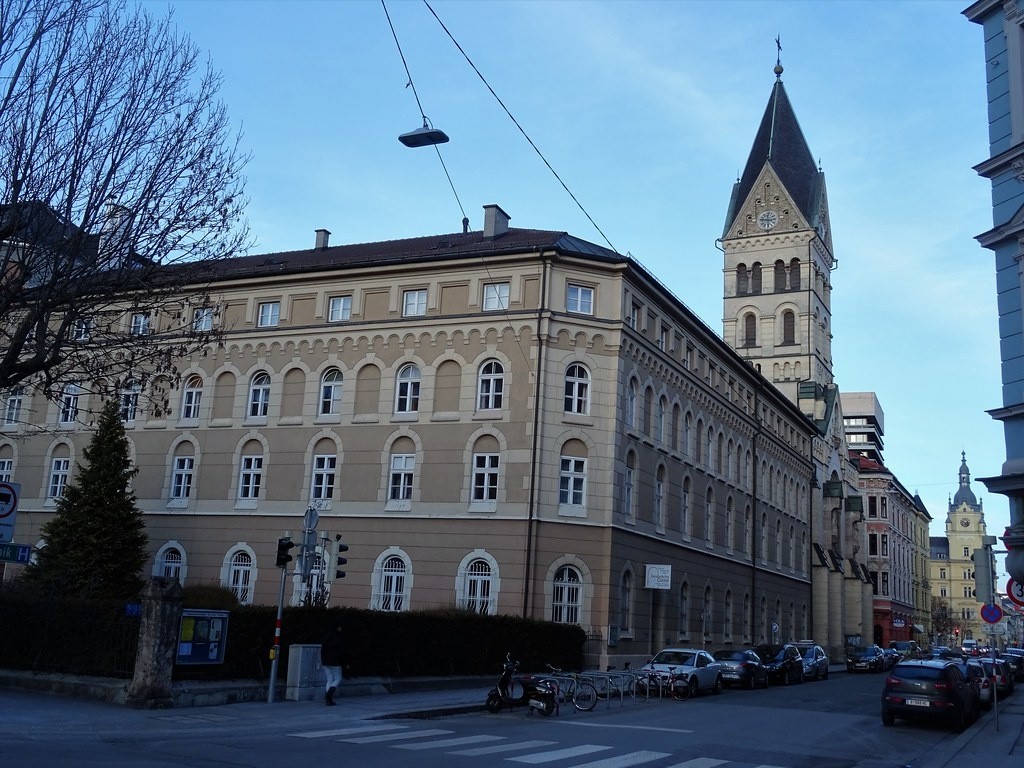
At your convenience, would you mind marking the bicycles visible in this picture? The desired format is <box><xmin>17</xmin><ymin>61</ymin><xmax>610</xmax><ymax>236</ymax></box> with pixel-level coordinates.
<box><xmin>543</xmin><ymin>658</ymin><xmax>598</xmax><ymax>713</ymax></box>
<box><xmin>638</xmin><ymin>663</ymin><xmax>692</xmax><ymax>702</ymax></box>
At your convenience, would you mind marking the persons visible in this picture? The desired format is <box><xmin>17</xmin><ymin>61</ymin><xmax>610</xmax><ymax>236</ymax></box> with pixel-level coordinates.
<box><xmin>320</xmin><ymin>622</ymin><xmax>350</xmax><ymax>706</ymax></box>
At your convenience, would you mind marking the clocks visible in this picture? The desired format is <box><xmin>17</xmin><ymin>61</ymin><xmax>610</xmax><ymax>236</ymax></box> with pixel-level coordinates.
<box><xmin>757</xmin><ymin>210</ymin><xmax>779</xmax><ymax>230</ymax></box>
<box><xmin>818</xmin><ymin>221</ymin><xmax>825</xmax><ymax>239</ymax></box>
<box><xmin>960</xmin><ymin>517</ymin><xmax>970</xmax><ymax>527</ymax></box>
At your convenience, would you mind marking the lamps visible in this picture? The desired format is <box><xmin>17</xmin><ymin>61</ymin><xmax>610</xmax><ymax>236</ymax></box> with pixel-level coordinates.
<box><xmin>813</xmin><ymin>543</ymin><xmax>831</xmax><ymax>568</ymax></box>
<box><xmin>860</xmin><ymin>563</ymin><xmax>876</xmax><ymax>586</ymax></box>
<box><xmin>848</xmin><ymin>559</ymin><xmax>865</xmax><ymax>580</ymax></box>
<box><xmin>828</xmin><ymin>549</ymin><xmax>845</xmax><ymax>574</ymax></box>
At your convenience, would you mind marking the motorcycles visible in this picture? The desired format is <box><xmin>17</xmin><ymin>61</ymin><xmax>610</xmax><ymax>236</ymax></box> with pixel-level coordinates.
<box><xmin>485</xmin><ymin>651</ymin><xmax>553</xmax><ymax>720</ymax></box>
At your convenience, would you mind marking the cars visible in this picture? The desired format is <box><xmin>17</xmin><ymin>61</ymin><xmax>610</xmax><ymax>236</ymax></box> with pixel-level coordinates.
<box><xmin>791</xmin><ymin>638</ymin><xmax>831</xmax><ymax>680</ymax></box>
<box><xmin>713</xmin><ymin>647</ymin><xmax>770</xmax><ymax>688</ymax></box>
<box><xmin>638</xmin><ymin>644</ymin><xmax>724</xmax><ymax>699</ymax></box>
<box><xmin>756</xmin><ymin>642</ymin><xmax>806</xmax><ymax>685</ymax></box>
<box><xmin>845</xmin><ymin>640</ymin><xmax>1024</xmax><ymax>732</ymax></box>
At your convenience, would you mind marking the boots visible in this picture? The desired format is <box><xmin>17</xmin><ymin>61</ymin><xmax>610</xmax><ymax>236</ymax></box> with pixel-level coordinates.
<box><xmin>325</xmin><ymin>686</ymin><xmax>336</xmax><ymax>706</ymax></box>
